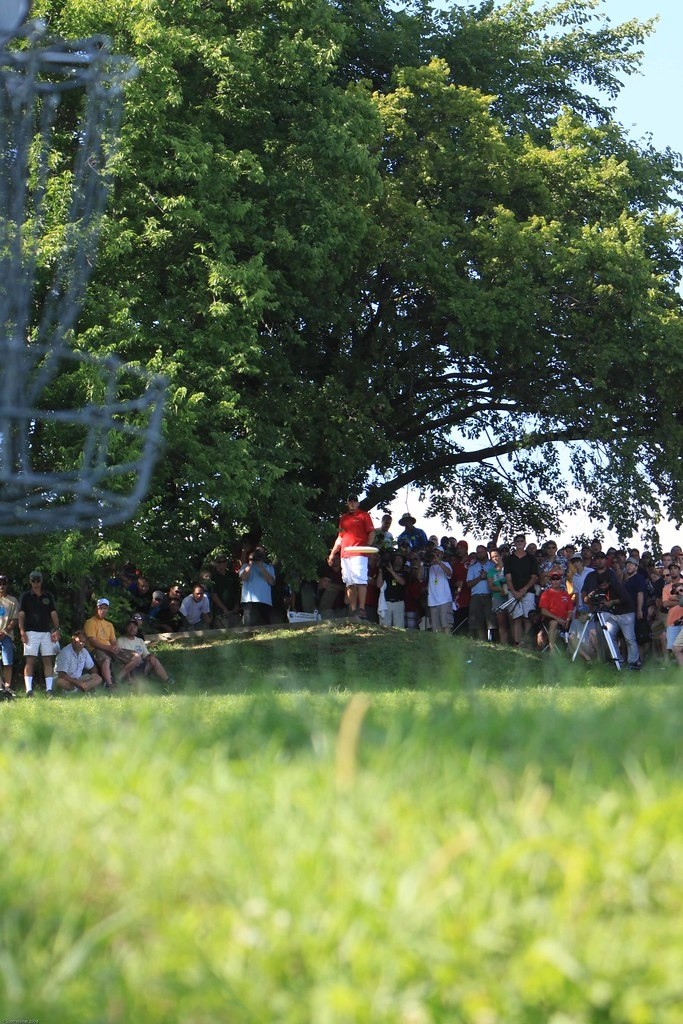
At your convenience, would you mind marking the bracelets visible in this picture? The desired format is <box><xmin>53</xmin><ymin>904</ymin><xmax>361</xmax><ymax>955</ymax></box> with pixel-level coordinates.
<box><xmin>438</xmin><ymin>562</ymin><xmax>442</xmax><ymax>565</ymax></box>
<box><xmin>610</xmin><ymin>600</ymin><xmax>614</xmax><ymax>605</ymax></box>
<box><xmin>379</xmin><ymin>571</ymin><xmax>382</xmax><ymax>573</ymax></box>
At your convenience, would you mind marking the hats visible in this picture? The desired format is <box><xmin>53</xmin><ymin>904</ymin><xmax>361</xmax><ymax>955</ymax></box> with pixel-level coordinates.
<box><xmin>550</xmin><ymin>571</ymin><xmax>561</xmax><ymax>578</ymax></box>
<box><xmin>514</xmin><ymin>535</ymin><xmax>525</xmax><ymax>541</ymax></box>
<box><xmin>625</xmin><ymin>557</ymin><xmax>637</xmax><ymax>565</ymax></box>
<box><xmin>393</xmin><ymin>549</ymin><xmax>406</xmax><ymax>560</ymax></box>
<box><xmin>670</xmin><ymin>583</ymin><xmax>683</xmax><ymax>595</ymax></box>
<box><xmin>570</xmin><ymin>554</ymin><xmax>583</xmax><ymax>561</ymax></box>
<box><xmin>0</xmin><ymin>575</ymin><xmax>9</xmax><ymax>582</ymax></box>
<box><xmin>399</xmin><ymin>513</ymin><xmax>416</xmax><ymax>526</ymax></box>
<box><xmin>594</xmin><ymin>551</ymin><xmax>607</xmax><ymax>558</ymax></box>
<box><xmin>97</xmin><ymin>598</ymin><xmax>109</xmax><ymax>607</ymax></box>
<box><xmin>432</xmin><ymin>546</ymin><xmax>444</xmax><ymax>553</ymax></box>
<box><xmin>30</xmin><ymin>571</ymin><xmax>42</xmax><ymax>579</ymax></box>
<box><xmin>348</xmin><ymin>495</ymin><xmax>358</xmax><ymax>502</ymax></box>
<box><xmin>126</xmin><ymin>618</ymin><xmax>138</xmax><ymax>626</ymax></box>
<box><xmin>668</xmin><ymin>561</ymin><xmax>681</xmax><ymax>570</ymax></box>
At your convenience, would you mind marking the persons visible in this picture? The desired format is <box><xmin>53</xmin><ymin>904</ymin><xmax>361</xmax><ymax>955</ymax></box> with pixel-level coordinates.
<box><xmin>83</xmin><ymin>598</ymin><xmax>142</xmax><ymax>692</ymax></box>
<box><xmin>447</xmin><ymin>533</ymin><xmax>683</xmax><ymax>672</ymax></box>
<box><xmin>133</xmin><ymin>611</ymin><xmax>145</xmax><ymax>640</ymax></box>
<box><xmin>107</xmin><ymin>567</ymin><xmax>232</xmax><ymax>633</ymax></box>
<box><xmin>17</xmin><ymin>571</ymin><xmax>61</xmax><ymax>698</ymax></box>
<box><xmin>53</xmin><ymin>630</ymin><xmax>103</xmax><ymax>693</ymax></box>
<box><xmin>328</xmin><ymin>494</ymin><xmax>376</xmax><ymax>619</ymax></box>
<box><xmin>115</xmin><ymin>618</ymin><xmax>176</xmax><ymax>687</ymax></box>
<box><xmin>0</xmin><ymin>574</ymin><xmax>21</xmax><ymax>695</ymax></box>
<box><xmin>238</xmin><ymin>549</ymin><xmax>276</xmax><ymax>626</ymax></box>
<box><xmin>312</xmin><ymin>552</ymin><xmax>350</xmax><ymax>620</ymax></box>
<box><xmin>345</xmin><ymin>512</ymin><xmax>455</xmax><ymax>635</ymax></box>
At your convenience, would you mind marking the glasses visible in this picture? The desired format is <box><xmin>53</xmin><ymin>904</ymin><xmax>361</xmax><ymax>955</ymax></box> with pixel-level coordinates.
<box><xmin>676</xmin><ymin>589</ymin><xmax>683</xmax><ymax>595</ymax></box>
<box><xmin>547</xmin><ymin>547</ymin><xmax>556</xmax><ymax>549</ymax></box>
<box><xmin>0</xmin><ymin>582</ymin><xmax>7</xmax><ymax>585</ymax></box>
<box><xmin>131</xmin><ymin>616</ymin><xmax>142</xmax><ymax>620</ymax></box>
<box><xmin>551</xmin><ymin>577</ymin><xmax>560</xmax><ymax>580</ymax></box>
<box><xmin>481</xmin><ymin>565</ymin><xmax>484</xmax><ymax>571</ymax></box>
<box><xmin>662</xmin><ymin>574</ymin><xmax>671</xmax><ymax>578</ymax></box>
<box><xmin>612</xmin><ymin>561</ymin><xmax>618</xmax><ymax>563</ymax></box>
<box><xmin>30</xmin><ymin>578</ymin><xmax>42</xmax><ymax>582</ymax></box>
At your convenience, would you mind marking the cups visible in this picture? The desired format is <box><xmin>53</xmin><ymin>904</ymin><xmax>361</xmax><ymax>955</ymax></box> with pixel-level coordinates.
<box><xmin>487</xmin><ymin>627</ymin><xmax>496</xmax><ymax>642</ymax></box>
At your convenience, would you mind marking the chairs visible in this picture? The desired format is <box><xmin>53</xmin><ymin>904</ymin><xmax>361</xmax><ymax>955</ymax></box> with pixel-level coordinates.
<box><xmin>74</xmin><ymin>629</ymin><xmax>122</xmax><ymax>693</ymax></box>
<box><xmin>534</xmin><ymin>595</ymin><xmax>572</xmax><ymax>654</ymax></box>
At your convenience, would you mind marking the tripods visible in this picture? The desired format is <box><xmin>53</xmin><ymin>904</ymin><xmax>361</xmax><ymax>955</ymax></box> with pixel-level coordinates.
<box><xmin>572</xmin><ymin>602</ymin><xmax>622</xmax><ymax>671</ymax></box>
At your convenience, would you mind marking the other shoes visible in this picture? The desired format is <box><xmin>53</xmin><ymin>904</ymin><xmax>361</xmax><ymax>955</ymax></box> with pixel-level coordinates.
<box><xmin>46</xmin><ymin>690</ymin><xmax>57</xmax><ymax>700</ymax></box>
<box><xmin>26</xmin><ymin>690</ymin><xmax>33</xmax><ymax>698</ymax></box>
<box><xmin>359</xmin><ymin>608</ymin><xmax>368</xmax><ymax>619</ymax></box>
<box><xmin>348</xmin><ymin>609</ymin><xmax>358</xmax><ymax>616</ymax></box>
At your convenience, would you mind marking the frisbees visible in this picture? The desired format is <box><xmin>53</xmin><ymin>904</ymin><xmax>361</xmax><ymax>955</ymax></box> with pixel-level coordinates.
<box><xmin>343</xmin><ymin>546</ymin><xmax>379</xmax><ymax>553</ymax></box>
